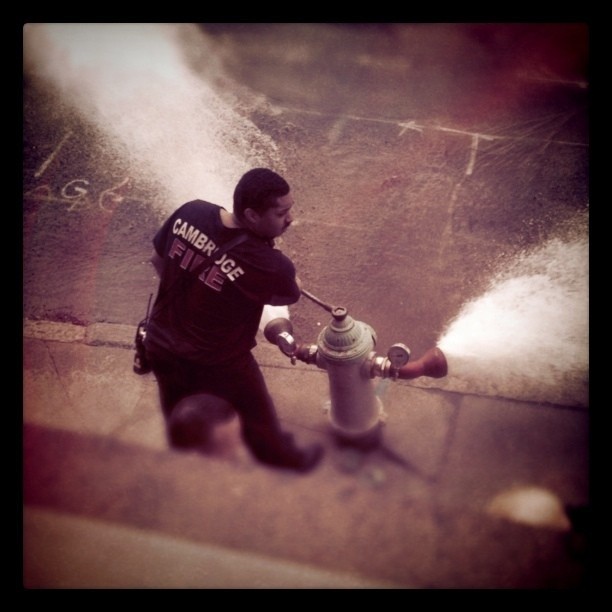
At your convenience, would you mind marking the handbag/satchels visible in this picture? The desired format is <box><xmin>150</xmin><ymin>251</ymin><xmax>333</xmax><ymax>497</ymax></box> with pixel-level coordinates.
<box><xmin>133</xmin><ymin>233</ymin><xmax>275</xmax><ymax>374</ymax></box>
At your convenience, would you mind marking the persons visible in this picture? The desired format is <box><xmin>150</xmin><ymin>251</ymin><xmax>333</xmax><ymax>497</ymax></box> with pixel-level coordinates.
<box><xmin>143</xmin><ymin>168</ymin><xmax>320</xmax><ymax>471</ymax></box>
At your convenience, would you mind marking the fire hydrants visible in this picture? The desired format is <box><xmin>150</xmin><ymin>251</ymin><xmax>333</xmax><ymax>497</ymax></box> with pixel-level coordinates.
<box><xmin>278</xmin><ymin>291</ymin><xmax>449</xmax><ymax>451</ymax></box>
